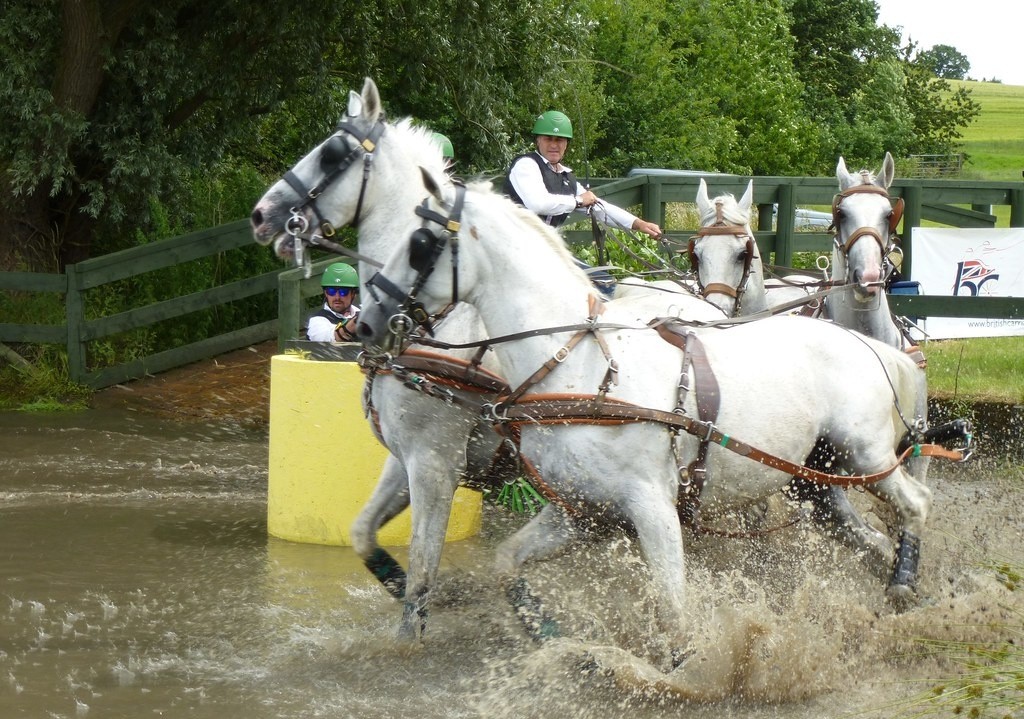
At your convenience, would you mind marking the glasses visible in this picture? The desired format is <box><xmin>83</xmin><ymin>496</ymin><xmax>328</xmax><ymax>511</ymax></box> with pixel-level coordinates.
<box><xmin>324</xmin><ymin>287</ymin><xmax>353</xmax><ymax>296</ymax></box>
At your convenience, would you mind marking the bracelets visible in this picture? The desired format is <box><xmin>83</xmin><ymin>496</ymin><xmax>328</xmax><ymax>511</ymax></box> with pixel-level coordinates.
<box><xmin>574</xmin><ymin>195</ymin><xmax>583</xmax><ymax>207</ymax></box>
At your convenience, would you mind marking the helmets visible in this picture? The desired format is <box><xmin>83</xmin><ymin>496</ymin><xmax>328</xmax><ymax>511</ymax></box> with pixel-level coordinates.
<box><xmin>320</xmin><ymin>263</ymin><xmax>359</xmax><ymax>288</ymax></box>
<box><xmin>431</xmin><ymin>133</ymin><xmax>454</xmax><ymax>159</ymax></box>
<box><xmin>532</xmin><ymin>111</ymin><xmax>573</xmax><ymax>139</ymax></box>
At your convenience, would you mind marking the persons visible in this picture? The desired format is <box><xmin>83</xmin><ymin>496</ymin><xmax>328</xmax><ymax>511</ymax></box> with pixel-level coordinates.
<box><xmin>308</xmin><ymin>263</ymin><xmax>358</xmax><ymax>342</ymax></box>
<box><xmin>505</xmin><ymin>111</ymin><xmax>663</xmax><ymax>240</ymax></box>
<box><xmin>432</xmin><ymin>132</ymin><xmax>453</xmax><ymax>172</ymax></box>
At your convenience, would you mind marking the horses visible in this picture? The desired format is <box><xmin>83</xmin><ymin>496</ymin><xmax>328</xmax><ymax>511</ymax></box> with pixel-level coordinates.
<box><xmin>684</xmin><ymin>175</ymin><xmax>828</xmax><ymax>335</ymax></box>
<box><xmin>826</xmin><ymin>152</ymin><xmax>927</xmax><ymax>401</ymax></box>
<box><xmin>351</xmin><ymin>166</ymin><xmax>933</xmax><ymax>659</ymax></box>
<box><xmin>251</xmin><ymin>73</ymin><xmax>529</xmax><ymax>653</ymax></box>
